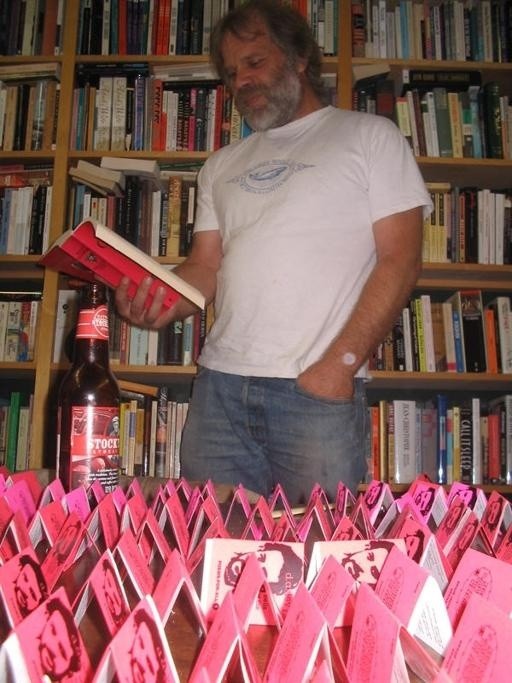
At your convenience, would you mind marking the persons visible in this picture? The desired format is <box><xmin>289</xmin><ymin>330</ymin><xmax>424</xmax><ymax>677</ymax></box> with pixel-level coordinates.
<box><xmin>38</xmin><ymin>597</ymin><xmax>82</xmax><ymax>680</ymax></box>
<box><xmin>10</xmin><ymin>551</ymin><xmax>50</xmax><ymax>615</ymax></box>
<box><xmin>126</xmin><ymin>606</ymin><xmax>168</xmax><ymax>681</ymax></box>
<box><xmin>334</xmin><ymin>538</ymin><xmax>399</xmax><ymax>587</ymax></box>
<box><xmin>269</xmin><ymin>481</ymin><xmax>512</xmax><ymax>564</ymax></box>
<box><xmin>96</xmin><ymin>558</ymin><xmax>125</xmax><ymax>626</ymax></box>
<box><xmin>113</xmin><ymin>1</ymin><xmax>434</xmax><ymax>505</ymax></box>
<box><xmin>221</xmin><ymin>540</ymin><xmax>304</xmax><ymax>595</ymax></box>
<box><xmin>51</xmin><ymin>519</ymin><xmax>85</xmax><ymax>569</ymax></box>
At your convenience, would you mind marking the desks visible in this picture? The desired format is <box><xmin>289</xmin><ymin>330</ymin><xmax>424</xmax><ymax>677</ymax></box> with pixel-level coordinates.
<box><xmin>0</xmin><ymin>484</ymin><xmax>511</xmax><ymax>682</ymax></box>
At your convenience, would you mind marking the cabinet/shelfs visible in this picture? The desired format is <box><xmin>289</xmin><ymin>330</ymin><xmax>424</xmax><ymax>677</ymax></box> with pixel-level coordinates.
<box><xmin>0</xmin><ymin>2</ymin><xmax>79</xmax><ymax>475</ymax></box>
<box><xmin>41</xmin><ymin>1</ymin><xmax>352</xmax><ymax>493</ymax></box>
<box><xmin>340</xmin><ymin>1</ymin><xmax>512</xmax><ymax>500</ymax></box>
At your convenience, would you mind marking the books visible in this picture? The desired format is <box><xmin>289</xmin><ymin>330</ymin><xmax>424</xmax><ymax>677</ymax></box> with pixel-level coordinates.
<box><xmin>254</xmin><ymin>501</ymin><xmax>350</xmax><ymax>518</ymax></box>
<box><xmin>1</xmin><ymin>0</ymin><xmax>510</xmax><ymax>488</ymax></box>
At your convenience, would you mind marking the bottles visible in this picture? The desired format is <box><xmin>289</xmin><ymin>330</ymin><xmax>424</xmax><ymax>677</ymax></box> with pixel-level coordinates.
<box><xmin>51</xmin><ymin>280</ymin><xmax>123</xmax><ymax>513</ymax></box>
